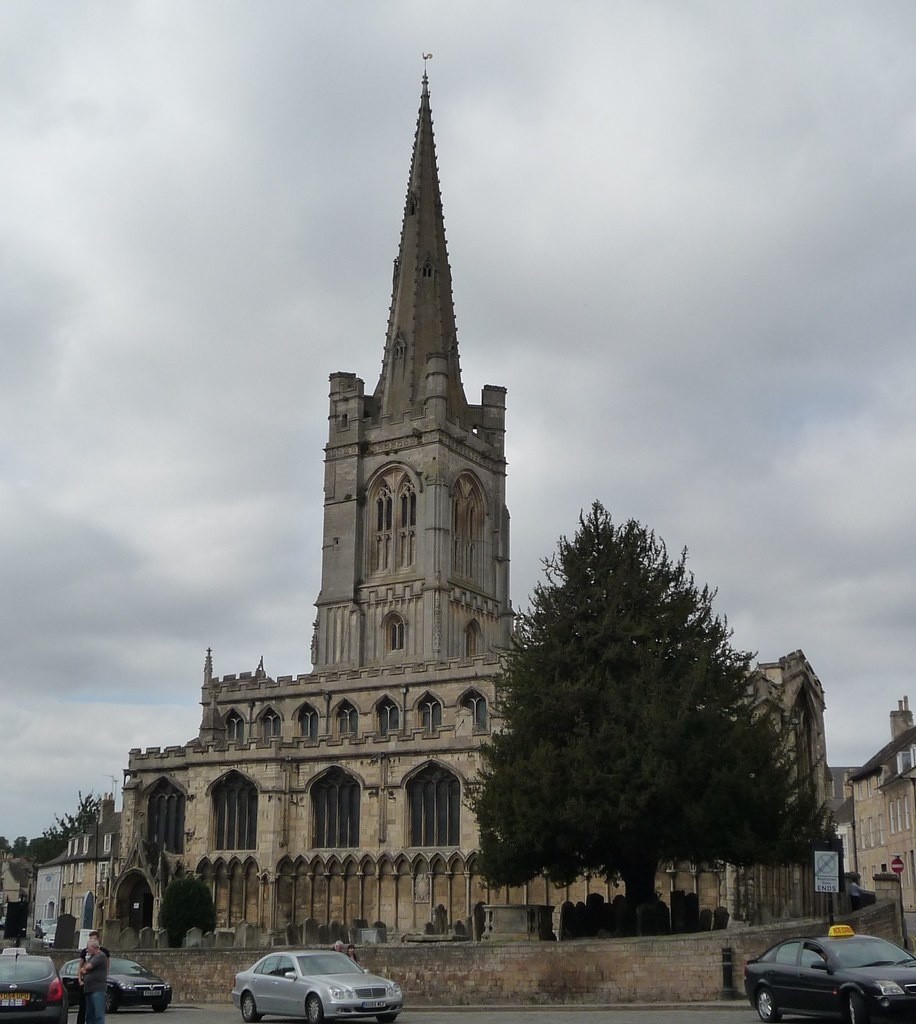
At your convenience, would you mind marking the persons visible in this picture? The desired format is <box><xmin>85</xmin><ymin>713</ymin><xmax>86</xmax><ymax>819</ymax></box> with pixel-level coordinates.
<box><xmin>77</xmin><ymin>931</ymin><xmax>110</xmax><ymax>1024</ymax></box>
<box><xmin>331</xmin><ymin>940</ymin><xmax>357</xmax><ymax>964</ymax></box>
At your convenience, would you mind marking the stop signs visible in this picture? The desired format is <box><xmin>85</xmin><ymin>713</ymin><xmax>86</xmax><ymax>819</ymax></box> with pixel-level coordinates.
<box><xmin>890</xmin><ymin>858</ymin><xmax>905</xmax><ymax>874</ymax></box>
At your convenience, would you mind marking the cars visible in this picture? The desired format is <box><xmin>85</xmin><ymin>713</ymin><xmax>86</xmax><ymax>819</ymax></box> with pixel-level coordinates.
<box><xmin>745</xmin><ymin>923</ymin><xmax>916</xmax><ymax>1024</ymax></box>
<box><xmin>230</xmin><ymin>950</ymin><xmax>405</xmax><ymax>1024</ymax></box>
<box><xmin>58</xmin><ymin>954</ymin><xmax>172</xmax><ymax>1013</ymax></box>
<box><xmin>0</xmin><ymin>947</ymin><xmax>69</xmax><ymax>1024</ymax></box>
<box><xmin>41</xmin><ymin>924</ymin><xmax>57</xmax><ymax>946</ymax></box>
